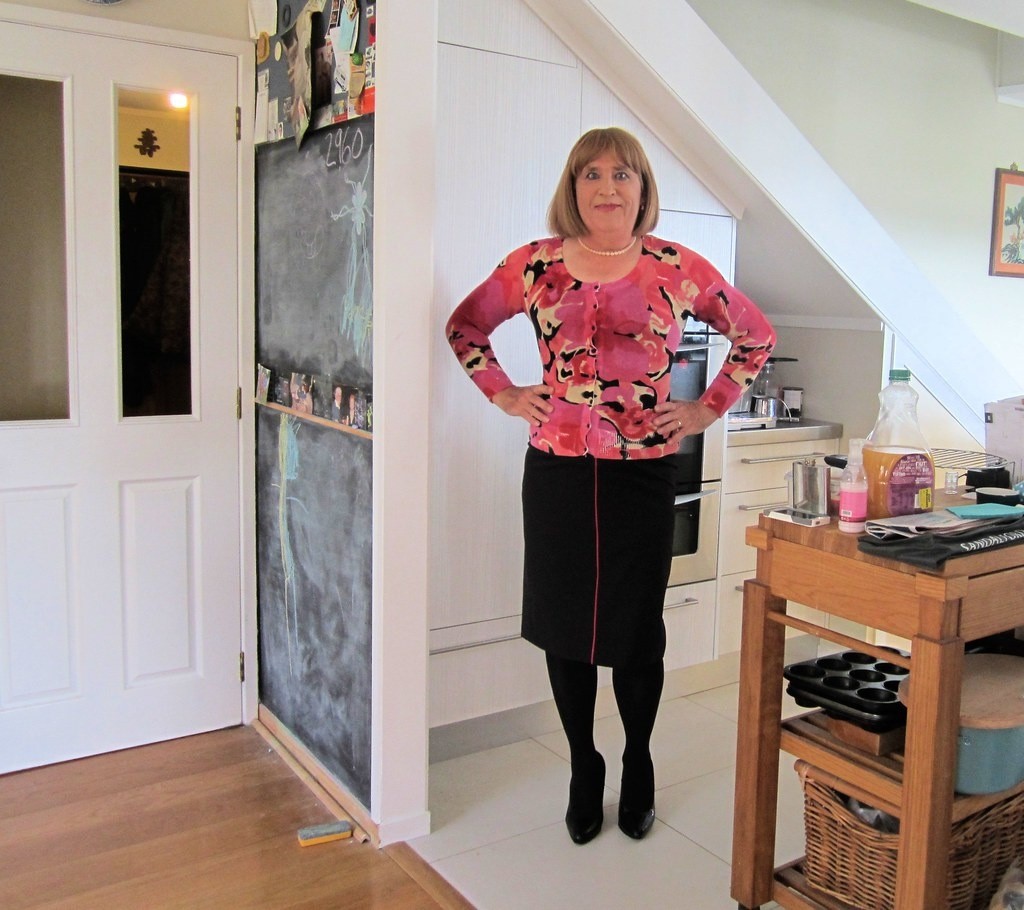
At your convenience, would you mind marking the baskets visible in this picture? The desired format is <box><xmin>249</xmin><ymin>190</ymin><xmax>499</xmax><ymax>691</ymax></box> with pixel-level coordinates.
<box><xmin>803</xmin><ymin>780</ymin><xmax>1024</xmax><ymax>910</ymax></box>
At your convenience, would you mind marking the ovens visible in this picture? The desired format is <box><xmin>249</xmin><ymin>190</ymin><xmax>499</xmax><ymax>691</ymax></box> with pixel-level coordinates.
<box><xmin>666</xmin><ymin>332</ymin><xmax>729</xmax><ymax>589</ymax></box>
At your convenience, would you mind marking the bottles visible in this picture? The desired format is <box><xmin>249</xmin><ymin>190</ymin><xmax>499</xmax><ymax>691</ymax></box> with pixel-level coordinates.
<box><xmin>751</xmin><ymin>361</ymin><xmax>781</xmax><ymax>418</ymax></box>
<box><xmin>860</xmin><ymin>369</ymin><xmax>935</xmax><ymax>520</ymax></box>
<box><xmin>838</xmin><ymin>439</ymin><xmax>868</xmax><ymax>534</ymax></box>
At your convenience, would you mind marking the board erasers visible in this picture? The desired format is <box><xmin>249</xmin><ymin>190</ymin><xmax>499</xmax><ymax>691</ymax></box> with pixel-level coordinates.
<box><xmin>297</xmin><ymin>820</ymin><xmax>352</xmax><ymax>847</ymax></box>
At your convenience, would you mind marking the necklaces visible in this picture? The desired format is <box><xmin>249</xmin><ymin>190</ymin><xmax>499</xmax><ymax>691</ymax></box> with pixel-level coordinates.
<box><xmin>577</xmin><ymin>236</ymin><xmax>637</xmax><ymax>256</ymax></box>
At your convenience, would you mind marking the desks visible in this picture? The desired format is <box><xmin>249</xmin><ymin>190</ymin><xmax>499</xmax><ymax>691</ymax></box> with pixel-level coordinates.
<box><xmin>731</xmin><ymin>487</ymin><xmax>1024</xmax><ymax>910</ymax></box>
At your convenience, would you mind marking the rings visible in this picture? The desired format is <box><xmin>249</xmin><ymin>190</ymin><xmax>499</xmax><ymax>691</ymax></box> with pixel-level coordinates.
<box><xmin>677</xmin><ymin>420</ymin><xmax>683</xmax><ymax>429</ymax></box>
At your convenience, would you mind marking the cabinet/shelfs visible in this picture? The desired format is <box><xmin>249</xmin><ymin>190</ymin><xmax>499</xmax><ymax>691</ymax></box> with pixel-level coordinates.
<box><xmin>718</xmin><ymin>439</ymin><xmax>841</xmax><ymax>657</ymax></box>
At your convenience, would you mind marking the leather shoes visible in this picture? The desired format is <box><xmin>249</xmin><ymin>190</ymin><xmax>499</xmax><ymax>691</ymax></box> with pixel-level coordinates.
<box><xmin>618</xmin><ymin>753</ymin><xmax>654</xmax><ymax>839</ymax></box>
<box><xmin>567</xmin><ymin>752</ymin><xmax>605</xmax><ymax>844</ymax></box>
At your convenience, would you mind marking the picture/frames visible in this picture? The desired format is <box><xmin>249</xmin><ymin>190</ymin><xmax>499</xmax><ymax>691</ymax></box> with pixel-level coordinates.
<box><xmin>987</xmin><ymin>168</ymin><xmax>1024</xmax><ymax>278</ymax></box>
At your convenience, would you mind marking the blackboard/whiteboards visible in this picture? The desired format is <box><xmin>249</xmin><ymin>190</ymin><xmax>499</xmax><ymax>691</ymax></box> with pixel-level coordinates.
<box><xmin>252</xmin><ymin>112</ymin><xmax>375</xmax><ymax>440</ymax></box>
<box><xmin>255</xmin><ymin>402</ymin><xmax>373</xmax><ymax>815</ymax></box>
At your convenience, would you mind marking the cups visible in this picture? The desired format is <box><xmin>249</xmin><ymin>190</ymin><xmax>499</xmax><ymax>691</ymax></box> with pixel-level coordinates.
<box><xmin>975</xmin><ymin>487</ymin><xmax>1019</xmax><ymax>507</ymax></box>
<box><xmin>754</xmin><ymin>398</ymin><xmax>777</xmax><ymax>417</ymax></box>
<box><xmin>966</xmin><ymin>467</ymin><xmax>1010</xmax><ymax>489</ymax></box>
<box><xmin>793</xmin><ymin>461</ymin><xmax>827</xmax><ymax>516</ymax></box>
<box><xmin>944</xmin><ymin>471</ymin><xmax>959</xmax><ymax>494</ymax></box>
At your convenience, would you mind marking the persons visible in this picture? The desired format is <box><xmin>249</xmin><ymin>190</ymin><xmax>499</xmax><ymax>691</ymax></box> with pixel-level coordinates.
<box><xmin>446</xmin><ymin>128</ymin><xmax>777</xmax><ymax>846</ymax></box>
